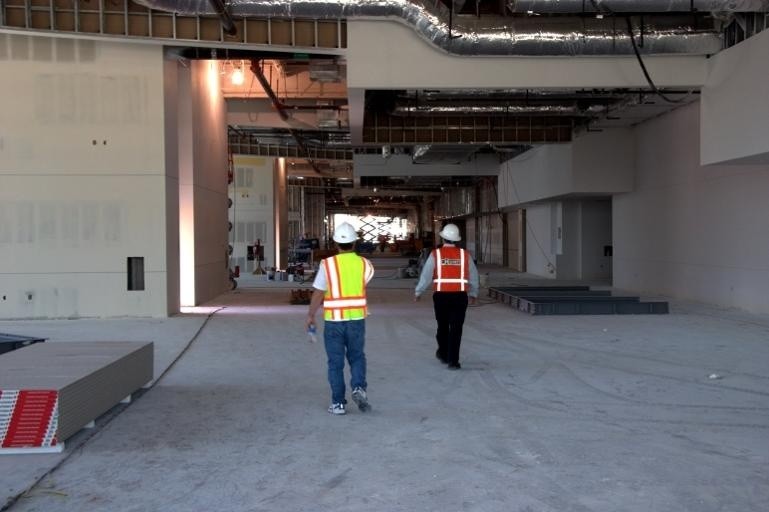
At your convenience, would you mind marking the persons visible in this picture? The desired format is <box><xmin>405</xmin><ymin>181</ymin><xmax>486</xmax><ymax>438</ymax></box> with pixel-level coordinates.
<box><xmin>413</xmin><ymin>223</ymin><xmax>481</xmax><ymax>369</ymax></box>
<box><xmin>303</xmin><ymin>222</ymin><xmax>375</xmax><ymax>415</ymax></box>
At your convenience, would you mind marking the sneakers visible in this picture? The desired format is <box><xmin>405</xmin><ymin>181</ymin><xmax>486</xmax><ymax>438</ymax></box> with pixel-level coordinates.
<box><xmin>351</xmin><ymin>386</ymin><xmax>372</xmax><ymax>412</ymax></box>
<box><xmin>327</xmin><ymin>402</ymin><xmax>346</xmax><ymax>414</ymax></box>
<box><xmin>436</xmin><ymin>352</ymin><xmax>460</xmax><ymax>370</ymax></box>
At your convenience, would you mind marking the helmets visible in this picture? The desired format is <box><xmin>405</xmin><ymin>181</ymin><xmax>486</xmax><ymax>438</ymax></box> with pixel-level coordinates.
<box><xmin>439</xmin><ymin>224</ymin><xmax>462</xmax><ymax>241</ymax></box>
<box><xmin>333</xmin><ymin>222</ymin><xmax>361</xmax><ymax>244</ymax></box>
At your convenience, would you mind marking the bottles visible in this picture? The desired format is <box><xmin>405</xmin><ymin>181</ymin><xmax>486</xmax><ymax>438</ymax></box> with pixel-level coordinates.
<box><xmin>307</xmin><ymin>321</ymin><xmax>318</xmax><ymax>344</ymax></box>
